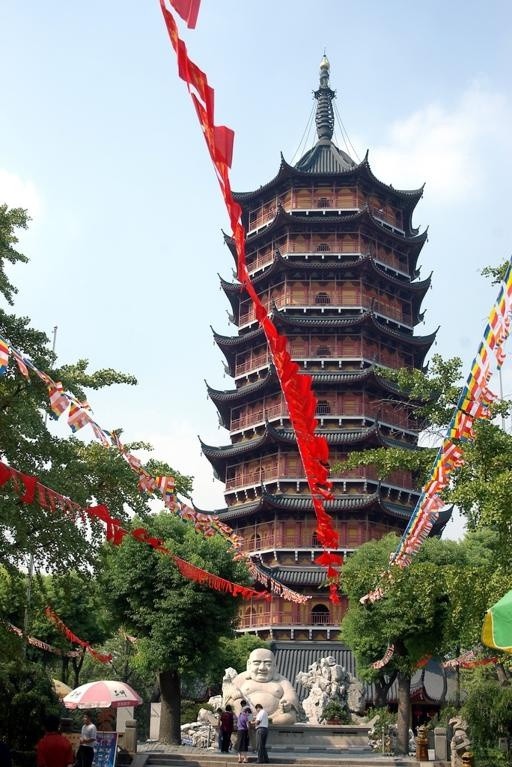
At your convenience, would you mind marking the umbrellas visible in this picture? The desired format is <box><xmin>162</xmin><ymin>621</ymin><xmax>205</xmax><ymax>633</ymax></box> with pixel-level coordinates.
<box><xmin>62</xmin><ymin>679</ymin><xmax>146</xmax><ymax>737</ymax></box>
<box><xmin>48</xmin><ymin>675</ymin><xmax>75</xmax><ymax>700</ymax></box>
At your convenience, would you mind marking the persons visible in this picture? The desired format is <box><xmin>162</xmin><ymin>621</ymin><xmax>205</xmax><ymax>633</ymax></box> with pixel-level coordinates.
<box><xmin>72</xmin><ymin>708</ymin><xmax>99</xmax><ymax>766</ymax></box>
<box><xmin>238</xmin><ymin>699</ymin><xmax>254</xmax><ymax>749</ymax></box>
<box><xmin>219</xmin><ymin>704</ymin><xmax>234</xmax><ymax>752</ymax></box>
<box><xmin>249</xmin><ymin>703</ymin><xmax>271</xmax><ymax>763</ymax></box>
<box><xmin>36</xmin><ymin>713</ymin><xmax>77</xmax><ymax>766</ymax></box>
<box><xmin>216</xmin><ymin>708</ymin><xmax>234</xmax><ymax>751</ymax></box>
<box><xmin>225</xmin><ymin>646</ymin><xmax>300</xmax><ymax>724</ymax></box>
<box><xmin>237</xmin><ymin>707</ymin><xmax>253</xmax><ymax>763</ymax></box>
